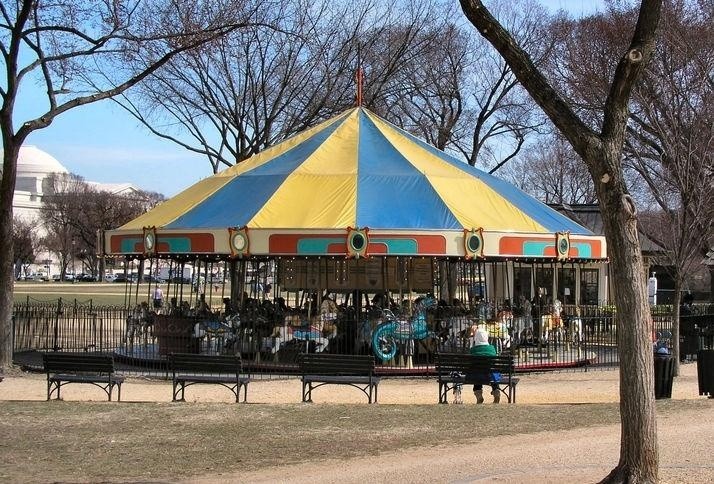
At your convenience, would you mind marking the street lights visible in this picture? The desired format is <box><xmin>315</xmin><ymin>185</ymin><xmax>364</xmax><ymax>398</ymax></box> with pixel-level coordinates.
<box><xmin>71</xmin><ymin>233</ymin><xmax>76</xmax><ymax>276</ymax></box>
<box><xmin>80</xmin><ymin>248</ymin><xmax>87</xmax><ymax>274</ymax></box>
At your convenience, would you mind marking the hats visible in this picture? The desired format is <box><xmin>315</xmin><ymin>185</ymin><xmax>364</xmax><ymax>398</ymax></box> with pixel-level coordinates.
<box><xmin>475</xmin><ymin>327</ymin><xmax>489</xmax><ymax>346</ymax></box>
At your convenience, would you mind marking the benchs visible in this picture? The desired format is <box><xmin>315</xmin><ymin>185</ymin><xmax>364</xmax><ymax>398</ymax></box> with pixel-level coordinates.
<box><xmin>434</xmin><ymin>351</ymin><xmax>520</xmax><ymax>404</ymax></box>
<box><xmin>296</xmin><ymin>350</ymin><xmax>381</xmax><ymax>404</ymax></box>
<box><xmin>168</xmin><ymin>350</ymin><xmax>251</xmax><ymax>403</ymax></box>
<box><xmin>41</xmin><ymin>349</ymin><xmax>125</xmax><ymax>401</ymax></box>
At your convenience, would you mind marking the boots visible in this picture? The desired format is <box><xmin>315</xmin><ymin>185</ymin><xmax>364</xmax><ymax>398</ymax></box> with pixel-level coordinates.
<box><xmin>491</xmin><ymin>389</ymin><xmax>501</xmax><ymax>404</ymax></box>
<box><xmin>474</xmin><ymin>389</ymin><xmax>484</xmax><ymax>404</ymax></box>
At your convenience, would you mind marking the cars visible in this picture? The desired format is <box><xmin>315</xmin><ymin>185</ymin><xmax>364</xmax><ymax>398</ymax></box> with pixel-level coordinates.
<box><xmin>17</xmin><ymin>261</ymin><xmax>278</xmax><ymax>284</ymax></box>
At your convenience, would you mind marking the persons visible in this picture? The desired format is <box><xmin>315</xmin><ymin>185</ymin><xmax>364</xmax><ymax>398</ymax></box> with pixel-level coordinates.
<box><xmin>265</xmin><ymin>279</ymin><xmax>272</xmax><ymax>302</ymax></box>
<box><xmin>197</xmin><ymin>273</ymin><xmax>204</xmax><ymax>292</ymax></box>
<box><xmin>150</xmin><ymin>282</ymin><xmax>164</xmax><ymax>314</ymax></box>
<box><xmin>192</xmin><ymin>274</ymin><xmax>198</xmax><ymax>292</ymax></box>
<box><xmin>464</xmin><ymin>326</ymin><xmax>500</xmax><ymax>403</ymax></box>
<box><xmin>252</xmin><ymin>278</ymin><xmax>263</xmax><ymax>298</ymax></box>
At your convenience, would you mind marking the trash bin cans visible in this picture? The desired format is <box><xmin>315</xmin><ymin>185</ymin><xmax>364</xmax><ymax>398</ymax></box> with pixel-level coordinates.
<box><xmin>697</xmin><ymin>349</ymin><xmax>714</xmax><ymax>399</ymax></box>
<box><xmin>653</xmin><ymin>353</ymin><xmax>677</xmax><ymax>400</ymax></box>
